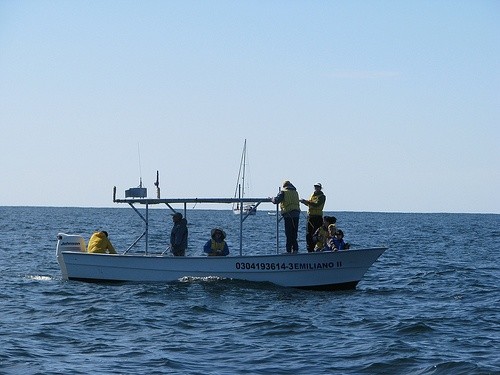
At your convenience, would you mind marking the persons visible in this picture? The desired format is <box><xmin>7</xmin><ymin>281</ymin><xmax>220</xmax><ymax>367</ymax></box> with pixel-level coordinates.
<box><xmin>169</xmin><ymin>213</ymin><xmax>188</xmax><ymax>256</ymax></box>
<box><xmin>202</xmin><ymin>226</ymin><xmax>230</xmax><ymax>256</ymax></box>
<box><xmin>271</xmin><ymin>180</ymin><xmax>301</xmax><ymax>253</ymax></box>
<box><xmin>88</xmin><ymin>231</ymin><xmax>117</xmax><ymax>254</ymax></box>
<box><xmin>302</xmin><ymin>183</ymin><xmax>326</xmax><ymax>253</ymax></box>
<box><xmin>313</xmin><ymin>217</ymin><xmax>349</xmax><ymax>251</ymax></box>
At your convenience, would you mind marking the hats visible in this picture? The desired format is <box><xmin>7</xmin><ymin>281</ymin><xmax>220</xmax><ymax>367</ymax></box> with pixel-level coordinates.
<box><xmin>314</xmin><ymin>183</ymin><xmax>322</xmax><ymax>189</ymax></box>
<box><xmin>211</xmin><ymin>228</ymin><xmax>226</xmax><ymax>239</ymax></box>
<box><xmin>170</xmin><ymin>213</ymin><xmax>183</xmax><ymax>220</ymax></box>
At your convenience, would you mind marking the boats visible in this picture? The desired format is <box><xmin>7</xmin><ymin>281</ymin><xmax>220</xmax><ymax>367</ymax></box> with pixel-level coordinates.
<box><xmin>55</xmin><ymin>232</ymin><xmax>388</xmax><ymax>288</ymax></box>
